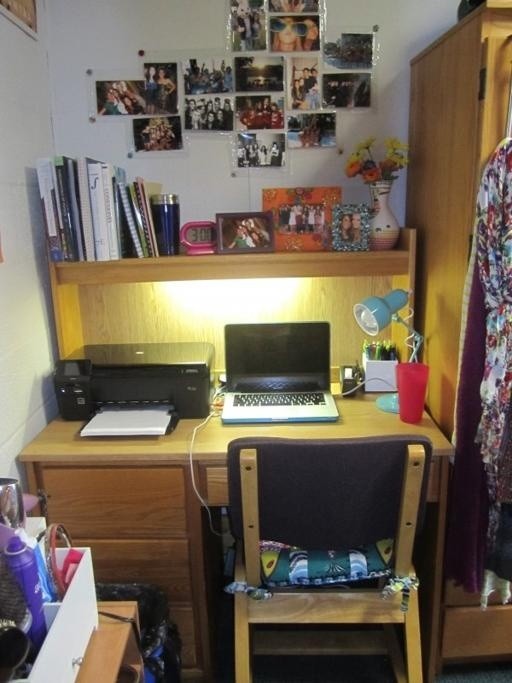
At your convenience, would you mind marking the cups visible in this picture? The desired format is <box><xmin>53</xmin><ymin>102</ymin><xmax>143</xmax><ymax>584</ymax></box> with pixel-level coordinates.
<box><xmin>397</xmin><ymin>361</ymin><xmax>430</xmax><ymax>425</ymax></box>
<box><xmin>149</xmin><ymin>193</ymin><xmax>181</xmax><ymax>256</ymax></box>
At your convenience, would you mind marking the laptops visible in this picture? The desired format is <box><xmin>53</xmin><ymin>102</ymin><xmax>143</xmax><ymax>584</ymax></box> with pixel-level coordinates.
<box><xmin>220</xmin><ymin>322</ymin><xmax>340</xmax><ymax>424</ymax></box>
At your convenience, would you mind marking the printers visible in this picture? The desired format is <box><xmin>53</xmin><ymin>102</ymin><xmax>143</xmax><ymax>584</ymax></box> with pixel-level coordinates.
<box><xmin>54</xmin><ymin>342</ymin><xmax>216</xmax><ymax>437</ymax></box>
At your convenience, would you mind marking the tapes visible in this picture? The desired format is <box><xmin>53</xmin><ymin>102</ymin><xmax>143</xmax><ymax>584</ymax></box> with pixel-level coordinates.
<box><xmin>344</xmin><ymin>367</ymin><xmax>353</xmax><ymax>379</ymax></box>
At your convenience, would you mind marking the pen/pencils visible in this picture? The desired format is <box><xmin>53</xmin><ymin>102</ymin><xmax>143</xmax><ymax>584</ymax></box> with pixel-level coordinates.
<box><xmin>362</xmin><ymin>338</ymin><xmax>396</xmax><ymax>360</ymax></box>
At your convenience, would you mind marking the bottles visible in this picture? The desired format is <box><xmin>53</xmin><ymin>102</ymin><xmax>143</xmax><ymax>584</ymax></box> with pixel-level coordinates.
<box><xmin>4</xmin><ymin>537</ymin><xmax>48</xmax><ymax>649</ymax></box>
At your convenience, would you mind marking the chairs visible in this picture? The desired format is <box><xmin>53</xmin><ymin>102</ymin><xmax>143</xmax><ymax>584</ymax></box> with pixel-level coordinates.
<box><xmin>221</xmin><ymin>434</ymin><xmax>433</xmax><ymax>682</ymax></box>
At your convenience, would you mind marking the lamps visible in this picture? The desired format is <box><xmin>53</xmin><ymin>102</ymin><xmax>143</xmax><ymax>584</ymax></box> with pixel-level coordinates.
<box><xmin>352</xmin><ymin>288</ymin><xmax>425</xmax><ymax>415</ymax></box>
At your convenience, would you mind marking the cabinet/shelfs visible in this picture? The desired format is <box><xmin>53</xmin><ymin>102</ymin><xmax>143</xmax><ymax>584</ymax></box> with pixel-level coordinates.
<box><xmin>405</xmin><ymin>1</ymin><xmax>511</xmax><ymax>680</ymax></box>
<box><xmin>78</xmin><ymin>601</ymin><xmax>147</xmax><ymax>682</ymax></box>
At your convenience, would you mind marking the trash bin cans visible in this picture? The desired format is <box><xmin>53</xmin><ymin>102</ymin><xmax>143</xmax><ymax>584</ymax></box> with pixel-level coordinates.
<box><xmin>95</xmin><ymin>582</ymin><xmax>182</xmax><ymax>683</ymax></box>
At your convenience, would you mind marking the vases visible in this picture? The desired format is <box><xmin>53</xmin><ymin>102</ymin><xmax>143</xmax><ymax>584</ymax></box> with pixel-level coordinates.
<box><xmin>367</xmin><ymin>180</ymin><xmax>400</xmax><ymax>249</ymax></box>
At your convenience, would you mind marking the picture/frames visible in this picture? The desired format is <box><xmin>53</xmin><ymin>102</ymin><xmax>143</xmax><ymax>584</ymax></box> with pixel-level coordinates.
<box><xmin>331</xmin><ymin>202</ymin><xmax>370</xmax><ymax>250</ymax></box>
<box><xmin>215</xmin><ymin>211</ymin><xmax>276</xmax><ymax>254</ymax></box>
<box><xmin>261</xmin><ymin>186</ymin><xmax>342</xmax><ymax>252</ymax></box>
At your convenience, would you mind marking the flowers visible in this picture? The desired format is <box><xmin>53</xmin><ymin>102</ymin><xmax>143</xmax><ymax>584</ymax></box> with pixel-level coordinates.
<box><xmin>341</xmin><ymin>135</ymin><xmax>410</xmax><ymax>187</ymax></box>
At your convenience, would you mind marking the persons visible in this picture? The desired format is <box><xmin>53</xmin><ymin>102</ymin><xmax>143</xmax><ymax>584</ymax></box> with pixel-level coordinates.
<box><xmin>232</xmin><ymin>0</ymin><xmax>261</xmax><ymax>52</ymax></box>
<box><xmin>270</xmin><ymin>16</ymin><xmax>318</xmax><ymax>51</ymax></box>
<box><xmin>98</xmin><ymin>59</ymin><xmax>318</xmax><ymax>167</ymax></box>
<box><xmin>278</xmin><ymin>203</ymin><xmax>325</xmax><ymax>235</ymax></box>
<box><xmin>228</xmin><ymin>216</ymin><xmax>270</xmax><ymax>249</ymax></box>
<box><xmin>340</xmin><ymin>214</ymin><xmax>355</xmax><ymax>242</ymax></box>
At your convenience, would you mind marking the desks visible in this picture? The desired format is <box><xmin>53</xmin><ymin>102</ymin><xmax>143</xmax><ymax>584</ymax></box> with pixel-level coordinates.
<box><xmin>20</xmin><ymin>226</ymin><xmax>454</xmax><ymax>681</ymax></box>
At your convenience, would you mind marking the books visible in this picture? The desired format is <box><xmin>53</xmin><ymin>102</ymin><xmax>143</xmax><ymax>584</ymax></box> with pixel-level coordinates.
<box><xmin>36</xmin><ymin>154</ymin><xmax>164</xmax><ymax>263</ymax></box>
<box><xmin>80</xmin><ymin>404</ymin><xmax>175</xmax><ymax>437</ymax></box>
